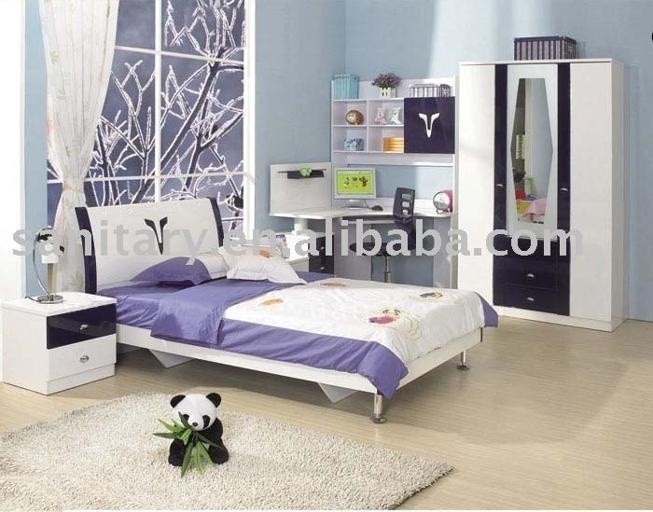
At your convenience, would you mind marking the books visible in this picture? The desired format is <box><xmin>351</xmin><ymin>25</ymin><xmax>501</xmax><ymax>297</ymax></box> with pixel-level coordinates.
<box><xmin>335</xmin><ymin>73</ymin><xmax>358</xmax><ymax>99</ymax></box>
<box><xmin>408</xmin><ymin>83</ymin><xmax>450</xmax><ymax>97</ymax></box>
<box><xmin>382</xmin><ymin>137</ymin><xmax>404</xmax><ymax>152</ymax></box>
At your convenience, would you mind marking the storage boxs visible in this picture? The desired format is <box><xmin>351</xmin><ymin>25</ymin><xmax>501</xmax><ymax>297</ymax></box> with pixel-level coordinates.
<box><xmin>514</xmin><ymin>36</ymin><xmax>577</xmax><ymax>61</ymax></box>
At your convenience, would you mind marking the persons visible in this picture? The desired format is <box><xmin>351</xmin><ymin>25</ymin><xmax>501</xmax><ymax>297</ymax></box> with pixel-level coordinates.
<box><xmin>168</xmin><ymin>392</ymin><xmax>228</xmax><ymax>466</ymax></box>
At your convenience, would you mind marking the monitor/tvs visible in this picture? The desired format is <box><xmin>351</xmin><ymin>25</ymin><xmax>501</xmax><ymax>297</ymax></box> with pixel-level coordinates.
<box><xmin>333</xmin><ymin>167</ymin><xmax>376</xmax><ymax>209</ymax></box>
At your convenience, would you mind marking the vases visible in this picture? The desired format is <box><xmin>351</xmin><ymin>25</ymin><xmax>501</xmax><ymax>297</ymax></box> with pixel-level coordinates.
<box><xmin>378</xmin><ymin>87</ymin><xmax>396</xmax><ymax>98</ymax></box>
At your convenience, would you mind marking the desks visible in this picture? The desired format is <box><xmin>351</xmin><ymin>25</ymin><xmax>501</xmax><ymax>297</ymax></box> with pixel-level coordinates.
<box><xmin>271</xmin><ymin>197</ymin><xmax>457</xmax><ymax>291</ymax></box>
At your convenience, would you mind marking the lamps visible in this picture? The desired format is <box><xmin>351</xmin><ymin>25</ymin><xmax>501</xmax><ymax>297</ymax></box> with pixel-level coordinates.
<box><xmin>24</xmin><ymin>224</ymin><xmax>65</xmax><ymax>305</ymax></box>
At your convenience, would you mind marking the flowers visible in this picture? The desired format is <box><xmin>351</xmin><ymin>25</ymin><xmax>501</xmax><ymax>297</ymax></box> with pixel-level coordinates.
<box><xmin>371</xmin><ymin>72</ymin><xmax>402</xmax><ymax>91</ymax></box>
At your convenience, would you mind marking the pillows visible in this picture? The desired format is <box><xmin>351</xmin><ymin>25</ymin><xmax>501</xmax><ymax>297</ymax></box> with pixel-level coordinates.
<box><xmin>129</xmin><ymin>241</ymin><xmax>308</xmax><ymax>294</ymax></box>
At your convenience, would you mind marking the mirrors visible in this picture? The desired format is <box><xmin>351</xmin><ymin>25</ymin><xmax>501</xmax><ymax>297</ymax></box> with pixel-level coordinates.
<box><xmin>506</xmin><ymin>64</ymin><xmax>558</xmax><ymax>240</ymax></box>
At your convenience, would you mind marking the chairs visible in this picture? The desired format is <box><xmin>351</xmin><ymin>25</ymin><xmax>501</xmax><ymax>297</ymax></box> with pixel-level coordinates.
<box><xmin>348</xmin><ymin>186</ymin><xmax>417</xmax><ymax>282</ymax></box>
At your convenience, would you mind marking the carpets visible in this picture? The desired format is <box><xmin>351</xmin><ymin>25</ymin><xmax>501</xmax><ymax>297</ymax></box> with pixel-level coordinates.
<box><xmin>0</xmin><ymin>390</ymin><xmax>455</xmax><ymax>511</ymax></box>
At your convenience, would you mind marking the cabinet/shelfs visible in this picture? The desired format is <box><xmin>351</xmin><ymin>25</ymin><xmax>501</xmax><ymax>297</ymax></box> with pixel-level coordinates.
<box><xmin>456</xmin><ymin>56</ymin><xmax>631</xmax><ymax>333</ymax></box>
<box><xmin>330</xmin><ymin>78</ymin><xmax>455</xmax><ymax>167</ymax></box>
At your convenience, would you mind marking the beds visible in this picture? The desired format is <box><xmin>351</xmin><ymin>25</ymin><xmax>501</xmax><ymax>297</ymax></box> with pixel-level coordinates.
<box><xmin>74</xmin><ymin>197</ymin><xmax>481</xmax><ymax>423</ymax></box>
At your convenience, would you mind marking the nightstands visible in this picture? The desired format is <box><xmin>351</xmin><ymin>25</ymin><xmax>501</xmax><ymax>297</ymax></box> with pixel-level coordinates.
<box><xmin>1</xmin><ymin>290</ymin><xmax>117</xmax><ymax>397</ymax></box>
<box><xmin>278</xmin><ymin>243</ymin><xmax>311</xmax><ymax>272</ymax></box>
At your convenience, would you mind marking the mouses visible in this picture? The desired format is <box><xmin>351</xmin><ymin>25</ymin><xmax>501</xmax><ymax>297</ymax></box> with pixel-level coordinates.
<box><xmin>372</xmin><ymin>205</ymin><xmax>384</xmax><ymax>211</ymax></box>
